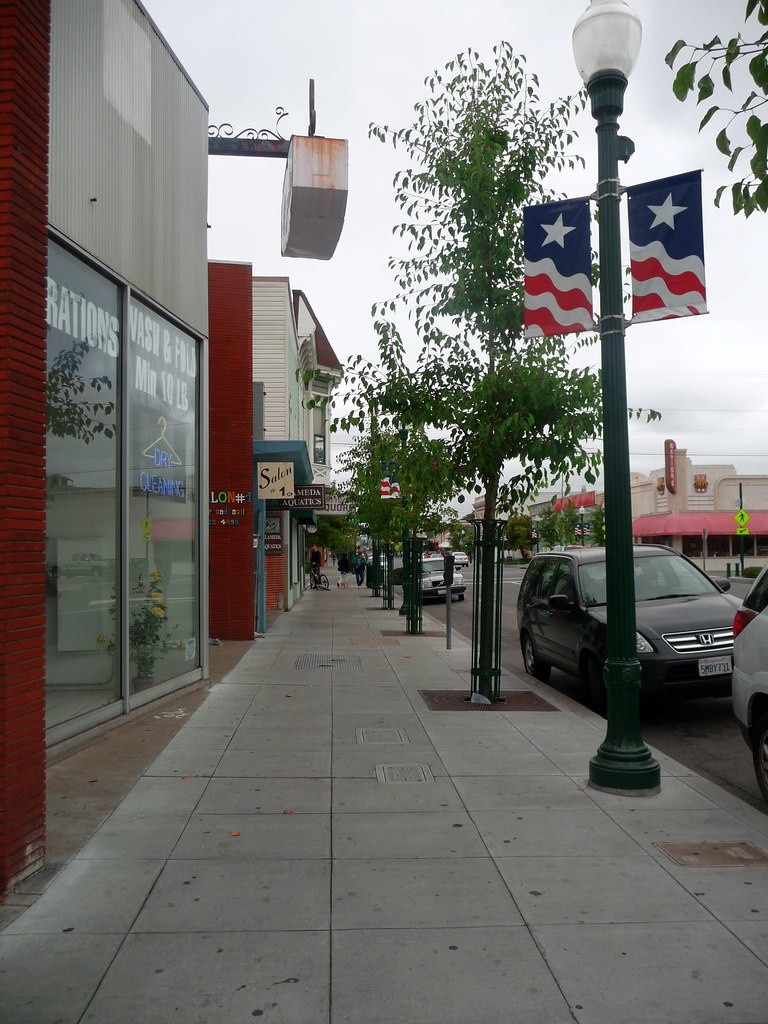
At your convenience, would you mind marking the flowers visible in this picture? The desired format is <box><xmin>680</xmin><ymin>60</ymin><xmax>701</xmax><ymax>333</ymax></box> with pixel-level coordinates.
<box><xmin>96</xmin><ymin>572</ymin><xmax>187</xmax><ymax>678</ymax></box>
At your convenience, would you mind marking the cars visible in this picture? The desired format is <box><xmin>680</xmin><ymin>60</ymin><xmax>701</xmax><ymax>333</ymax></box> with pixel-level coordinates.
<box><xmin>416</xmin><ymin>556</ymin><xmax>466</xmax><ymax>604</ymax></box>
<box><xmin>451</xmin><ymin>551</ymin><xmax>469</xmax><ymax>568</ymax></box>
<box><xmin>368</xmin><ymin>555</ymin><xmax>388</xmax><ymax>572</ymax></box>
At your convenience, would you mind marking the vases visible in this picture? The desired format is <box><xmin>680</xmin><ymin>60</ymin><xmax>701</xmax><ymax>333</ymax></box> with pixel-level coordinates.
<box><xmin>131</xmin><ymin>677</ymin><xmax>154</xmax><ymax>693</ymax></box>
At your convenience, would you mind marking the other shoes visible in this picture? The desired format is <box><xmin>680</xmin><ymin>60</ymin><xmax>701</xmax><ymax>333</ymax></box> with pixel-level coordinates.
<box><xmin>337</xmin><ymin>583</ymin><xmax>339</xmax><ymax>587</ymax></box>
<box><xmin>312</xmin><ymin>586</ymin><xmax>318</xmax><ymax>589</ymax></box>
<box><xmin>358</xmin><ymin>585</ymin><xmax>361</xmax><ymax>589</ymax></box>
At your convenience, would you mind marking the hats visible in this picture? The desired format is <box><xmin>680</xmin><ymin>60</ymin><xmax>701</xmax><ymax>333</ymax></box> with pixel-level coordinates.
<box><xmin>357</xmin><ymin>551</ymin><xmax>362</xmax><ymax>555</ymax></box>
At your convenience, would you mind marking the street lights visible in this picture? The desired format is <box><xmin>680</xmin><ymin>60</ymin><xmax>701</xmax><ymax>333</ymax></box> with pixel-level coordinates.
<box><xmin>571</xmin><ymin>0</ymin><xmax>664</xmax><ymax>801</ymax></box>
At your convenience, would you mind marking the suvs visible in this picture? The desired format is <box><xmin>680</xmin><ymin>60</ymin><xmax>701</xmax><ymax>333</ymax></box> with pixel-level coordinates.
<box><xmin>729</xmin><ymin>556</ymin><xmax>767</xmax><ymax>805</ymax></box>
<box><xmin>516</xmin><ymin>543</ymin><xmax>745</xmax><ymax>717</ymax></box>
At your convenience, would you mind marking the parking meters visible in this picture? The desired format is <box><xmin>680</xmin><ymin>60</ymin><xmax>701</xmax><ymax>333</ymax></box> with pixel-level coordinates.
<box><xmin>443</xmin><ymin>554</ymin><xmax>455</xmax><ymax>650</ymax></box>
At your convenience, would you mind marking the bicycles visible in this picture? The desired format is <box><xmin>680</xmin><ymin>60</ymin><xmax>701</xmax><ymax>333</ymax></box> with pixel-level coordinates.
<box><xmin>309</xmin><ymin>562</ymin><xmax>329</xmax><ymax>590</ymax></box>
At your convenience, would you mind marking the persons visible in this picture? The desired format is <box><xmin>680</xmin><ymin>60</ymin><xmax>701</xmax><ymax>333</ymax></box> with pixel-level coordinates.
<box><xmin>332</xmin><ymin>554</ymin><xmax>336</xmax><ymax>566</ymax></box>
<box><xmin>351</xmin><ymin>548</ymin><xmax>369</xmax><ymax>589</ymax></box>
<box><xmin>337</xmin><ymin>553</ymin><xmax>350</xmax><ymax>588</ymax></box>
<box><xmin>310</xmin><ymin>544</ymin><xmax>322</xmax><ymax>589</ymax></box>
